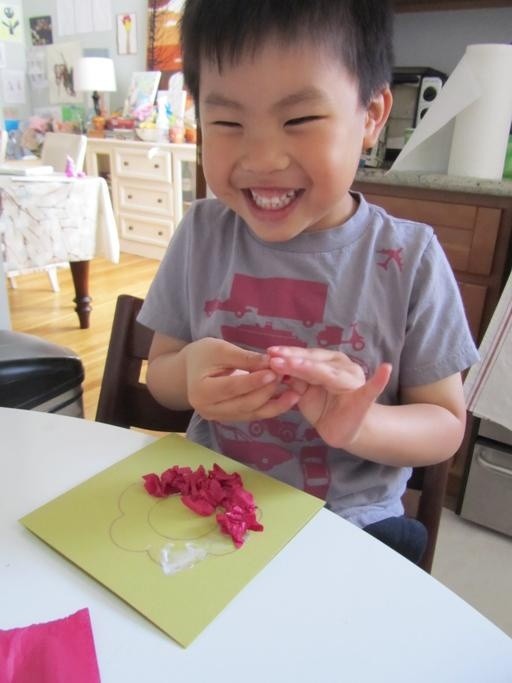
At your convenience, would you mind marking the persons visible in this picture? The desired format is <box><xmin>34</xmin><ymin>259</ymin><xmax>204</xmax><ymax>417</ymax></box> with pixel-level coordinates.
<box><xmin>135</xmin><ymin>0</ymin><xmax>480</xmax><ymax>565</ymax></box>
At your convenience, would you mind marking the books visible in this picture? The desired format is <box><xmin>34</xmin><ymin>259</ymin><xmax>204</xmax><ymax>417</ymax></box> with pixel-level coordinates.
<box><xmin>0</xmin><ymin>166</ymin><xmax>53</xmax><ymax>174</ymax></box>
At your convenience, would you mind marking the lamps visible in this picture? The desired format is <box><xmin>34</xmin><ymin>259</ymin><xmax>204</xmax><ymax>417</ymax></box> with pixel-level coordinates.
<box><xmin>73</xmin><ymin>57</ymin><xmax>119</xmax><ymax>116</ymax></box>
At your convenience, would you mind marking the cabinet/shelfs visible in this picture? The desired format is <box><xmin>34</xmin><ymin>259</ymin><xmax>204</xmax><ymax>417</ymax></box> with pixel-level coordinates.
<box><xmin>86</xmin><ymin>137</ymin><xmax>197</xmax><ymax>263</ymax></box>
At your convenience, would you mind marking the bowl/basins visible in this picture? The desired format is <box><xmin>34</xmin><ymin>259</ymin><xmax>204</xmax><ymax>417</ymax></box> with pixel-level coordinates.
<box><xmin>135</xmin><ymin>124</ymin><xmax>167</xmax><ymax>144</ymax></box>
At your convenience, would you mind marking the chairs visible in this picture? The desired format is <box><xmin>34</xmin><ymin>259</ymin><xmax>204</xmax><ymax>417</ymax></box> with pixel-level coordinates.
<box><xmin>92</xmin><ymin>288</ymin><xmax>447</xmax><ymax>573</ymax></box>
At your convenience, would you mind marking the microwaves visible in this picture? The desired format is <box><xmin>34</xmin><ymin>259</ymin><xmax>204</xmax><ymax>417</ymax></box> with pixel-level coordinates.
<box><xmin>388</xmin><ymin>63</ymin><xmax>450</xmax><ymax>154</ymax></box>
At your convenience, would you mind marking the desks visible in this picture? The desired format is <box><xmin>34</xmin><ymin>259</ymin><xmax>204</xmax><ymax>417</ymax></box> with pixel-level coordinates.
<box><xmin>346</xmin><ymin>166</ymin><xmax>511</xmax><ymax>517</ymax></box>
<box><xmin>0</xmin><ymin>406</ymin><xmax>512</xmax><ymax>683</ymax></box>
<box><xmin>0</xmin><ymin>158</ymin><xmax>124</xmax><ymax>332</ymax></box>
